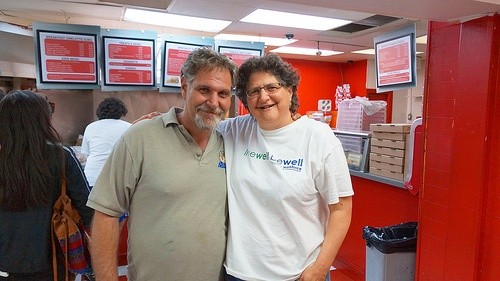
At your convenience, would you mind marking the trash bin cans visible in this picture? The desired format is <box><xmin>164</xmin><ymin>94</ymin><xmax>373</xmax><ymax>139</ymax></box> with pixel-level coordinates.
<box><xmin>363</xmin><ymin>221</ymin><xmax>417</xmax><ymax>281</ymax></box>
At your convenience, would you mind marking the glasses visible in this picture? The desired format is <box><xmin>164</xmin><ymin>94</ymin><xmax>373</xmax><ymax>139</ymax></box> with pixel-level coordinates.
<box><xmin>245</xmin><ymin>83</ymin><xmax>285</xmax><ymax>98</ymax></box>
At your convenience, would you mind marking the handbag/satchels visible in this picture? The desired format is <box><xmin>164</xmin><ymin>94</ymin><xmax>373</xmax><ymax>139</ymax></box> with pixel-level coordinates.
<box><xmin>51</xmin><ymin>144</ymin><xmax>94</xmax><ymax>281</ymax></box>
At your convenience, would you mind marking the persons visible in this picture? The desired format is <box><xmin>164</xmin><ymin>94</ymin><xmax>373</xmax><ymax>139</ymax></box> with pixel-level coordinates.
<box><xmin>132</xmin><ymin>51</ymin><xmax>355</xmax><ymax>281</ymax></box>
<box><xmin>86</xmin><ymin>47</ymin><xmax>237</xmax><ymax>281</ymax></box>
<box><xmin>74</xmin><ymin>98</ymin><xmax>132</xmax><ymax>281</ymax></box>
<box><xmin>0</xmin><ymin>90</ymin><xmax>93</xmax><ymax>281</ymax></box>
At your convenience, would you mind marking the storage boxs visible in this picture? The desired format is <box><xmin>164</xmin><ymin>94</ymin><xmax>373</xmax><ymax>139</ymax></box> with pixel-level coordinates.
<box><xmin>370</xmin><ymin>123</ymin><xmax>412</xmax><ymax>181</ymax></box>
